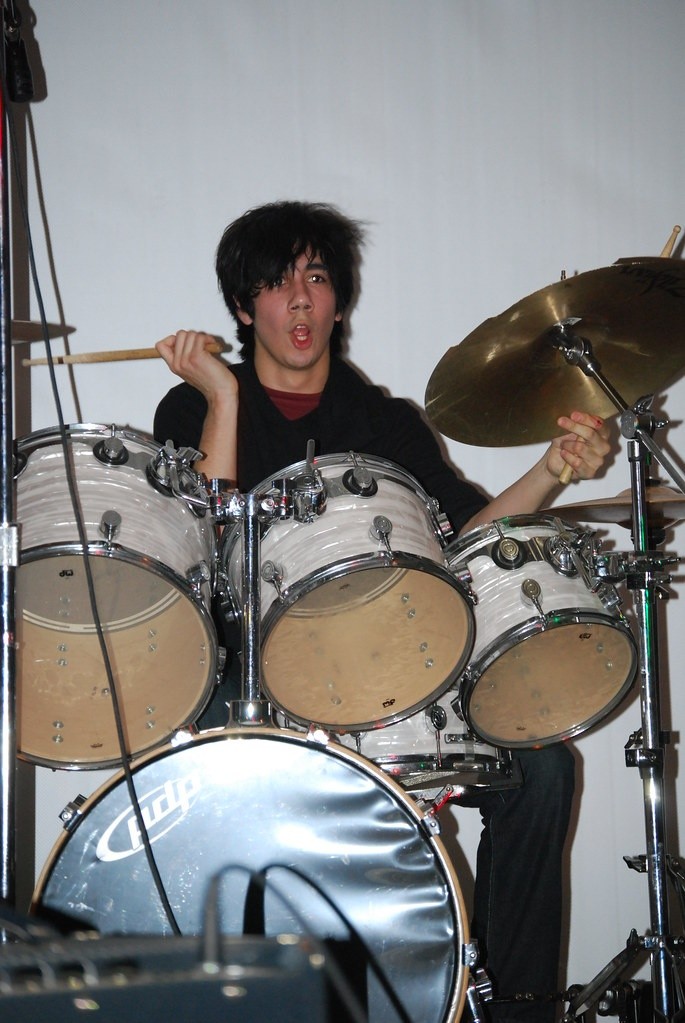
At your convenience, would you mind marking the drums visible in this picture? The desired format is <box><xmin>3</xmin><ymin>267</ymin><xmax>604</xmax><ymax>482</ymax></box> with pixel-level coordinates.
<box><xmin>25</xmin><ymin>725</ymin><xmax>473</xmax><ymax>1023</ymax></box>
<box><xmin>442</xmin><ymin>512</ymin><xmax>639</xmax><ymax>750</ymax></box>
<box><xmin>13</xmin><ymin>423</ymin><xmax>216</xmax><ymax>773</ymax></box>
<box><xmin>275</xmin><ymin>681</ymin><xmax>514</xmax><ymax>798</ymax></box>
<box><xmin>217</xmin><ymin>452</ymin><xmax>476</xmax><ymax>736</ymax></box>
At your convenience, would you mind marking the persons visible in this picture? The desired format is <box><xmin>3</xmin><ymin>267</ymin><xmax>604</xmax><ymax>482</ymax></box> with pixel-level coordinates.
<box><xmin>151</xmin><ymin>202</ymin><xmax>612</xmax><ymax>1023</ymax></box>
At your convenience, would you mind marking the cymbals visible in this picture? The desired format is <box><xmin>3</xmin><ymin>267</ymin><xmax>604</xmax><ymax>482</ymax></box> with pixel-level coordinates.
<box><xmin>422</xmin><ymin>257</ymin><xmax>685</xmax><ymax>448</ymax></box>
<box><xmin>538</xmin><ymin>483</ymin><xmax>685</xmax><ymax>531</ymax></box>
